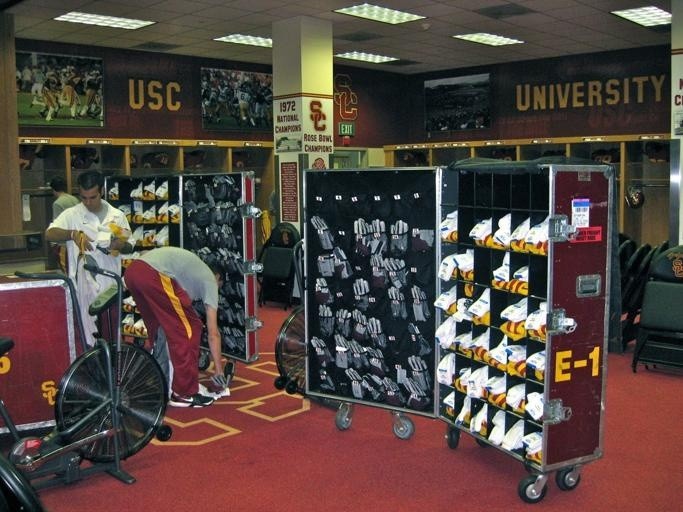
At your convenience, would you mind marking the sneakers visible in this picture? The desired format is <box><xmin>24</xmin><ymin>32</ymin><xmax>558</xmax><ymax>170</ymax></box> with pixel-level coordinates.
<box><xmin>170</xmin><ymin>393</ymin><xmax>214</xmax><ymax>409</ymax></box>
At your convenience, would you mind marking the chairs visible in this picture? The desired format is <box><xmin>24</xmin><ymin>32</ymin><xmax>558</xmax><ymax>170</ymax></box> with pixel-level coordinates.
<box><xmin>619</xmin><ymin>232</ymin><xmax>683</xmax><ymax>373</ymax></box>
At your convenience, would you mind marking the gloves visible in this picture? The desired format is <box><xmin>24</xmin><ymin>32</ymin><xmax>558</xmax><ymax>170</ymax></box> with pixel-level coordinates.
<box><xmin>309</xmin><ymin>216</ymin><xmax>431</xmax><ymax>401</ymax></box>
<box><xmin>183</xmin><ymin>176</ymin><xmax>245</xmax><ymax>352</ymax></box>
<box><xmin>211</xmin><ymin>375</ymin><xmax>231</xmax><ymax>396</ymax></box>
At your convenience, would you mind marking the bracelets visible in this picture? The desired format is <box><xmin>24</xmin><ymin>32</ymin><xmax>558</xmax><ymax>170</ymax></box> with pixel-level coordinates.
<box><xmin>70</xmin><ymin>230</ymin><xmax>74</xmax><ymax>241</ymax></box>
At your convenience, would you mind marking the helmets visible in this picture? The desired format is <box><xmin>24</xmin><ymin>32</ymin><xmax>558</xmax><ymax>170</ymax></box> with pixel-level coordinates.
<box><xmin>627</xmin><ymin>190</ymin><xmax>643</xmax><ymax>208</ymax></box>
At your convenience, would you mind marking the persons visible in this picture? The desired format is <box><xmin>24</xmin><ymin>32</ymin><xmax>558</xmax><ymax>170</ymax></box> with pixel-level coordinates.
<box><xmin>45</xmin><ymin>171</ymin><xmax>136</xmax><ymax>351</ymax></box>
<box><xmin>16</xmin><ymin>55</ymin><xmax>103</xmax><ymax>123</ymax></box>
<box><xmin>426</xmin><ymin>83</ymin><xmax>490</xmax><ymax>131</ymax></box>
<box><xmin>125</xmin><ymin>245</ymin><xmax>226</xmax><ymax>411</ymax></box>
<box><xmin>49</xmin><ymin>176</ymin><xmax>80</xmax><ymax>272</ymax></box>
<box><xmin>201</xmin><ymin>68</ymin><xmax>273</xmax><ymax>128</ymax></box>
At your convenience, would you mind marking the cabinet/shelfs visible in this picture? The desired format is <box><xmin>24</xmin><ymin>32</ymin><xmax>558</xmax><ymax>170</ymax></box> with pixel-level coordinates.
<box><xmin>104</xmin><ymin>171</ymin><xmax>264</xmax><ymax>379</ymax></box>
<box><xmin>303</xmin><ymin>163</ymin><xmax>614</xmax><ymax>472</ymax></box>
<box><xmin>18</xmin><ymin>133</ymin><xmax>672</xmax><ymax>259</ymax></box>
